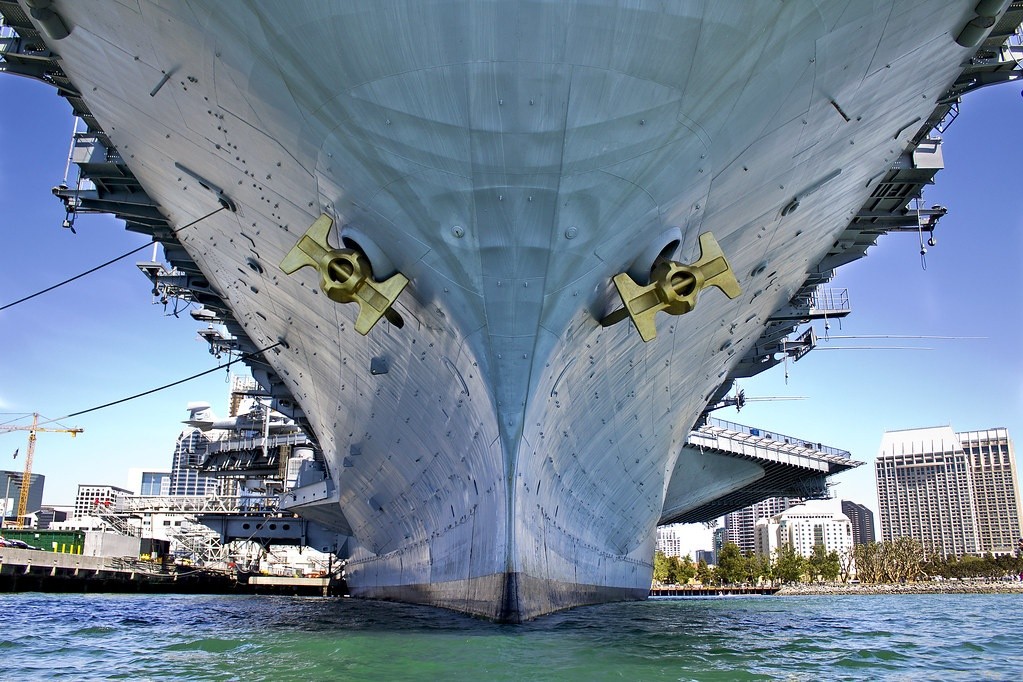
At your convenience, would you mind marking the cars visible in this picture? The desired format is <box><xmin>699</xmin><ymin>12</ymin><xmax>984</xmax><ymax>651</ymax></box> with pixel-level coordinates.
<box><xmin>0</xmin><ymin>536</ymin><xmax>36</xmax><ymax>550</ymax></box>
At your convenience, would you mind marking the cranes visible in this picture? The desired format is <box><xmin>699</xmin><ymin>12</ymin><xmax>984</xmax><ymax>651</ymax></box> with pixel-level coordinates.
<box><xmin>0</xmin><ymin>410</ymin><xmax>85</xmax><ymax>530</ymax></box>
